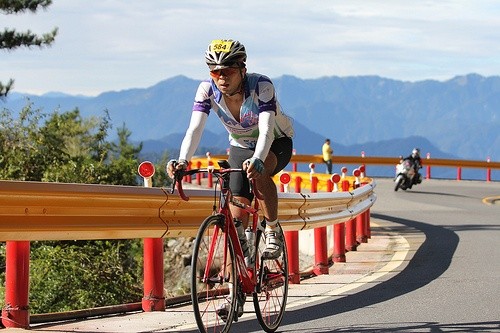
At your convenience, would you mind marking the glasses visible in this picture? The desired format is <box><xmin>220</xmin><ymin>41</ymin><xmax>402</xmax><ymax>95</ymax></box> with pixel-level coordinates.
<box><xmin>210</xmin><ymin>69</ymin><xmax>240</xmax><ymax>79</ymax></box>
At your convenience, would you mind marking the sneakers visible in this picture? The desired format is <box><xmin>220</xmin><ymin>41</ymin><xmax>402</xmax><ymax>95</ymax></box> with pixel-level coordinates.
<box><xmin>215</xmin><ymin>299</ymin><xmax>244</xmax><ymax>317</ymax></box>
<box><xmin>261</xmin><ymin>222</ymin><xmax>285</xmax><ymax>259</ymax></box>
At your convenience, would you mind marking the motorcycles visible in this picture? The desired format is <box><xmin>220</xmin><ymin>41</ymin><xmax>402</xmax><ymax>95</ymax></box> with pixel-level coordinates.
<box><xmin>392</xmin><ymin>155</ymin><xmax>422</xmax><ymax>191</ymax></box>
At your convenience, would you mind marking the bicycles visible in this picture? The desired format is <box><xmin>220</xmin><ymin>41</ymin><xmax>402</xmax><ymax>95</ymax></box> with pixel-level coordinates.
<box><xmin>166</xmin><ymin>155</ymin><xmax>291</xmax><ymax>333</ymax></box>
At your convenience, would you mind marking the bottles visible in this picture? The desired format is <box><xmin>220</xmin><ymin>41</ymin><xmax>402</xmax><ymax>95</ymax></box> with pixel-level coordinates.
<box><xmin>244</xmin><ymin>226</ymin><xmax>253</xmax><ymax>252</ymax></box>
<box><xmin>234</xmin><ymin>218</ymin><xmax>249</xmax><ymax>257</ymax></box>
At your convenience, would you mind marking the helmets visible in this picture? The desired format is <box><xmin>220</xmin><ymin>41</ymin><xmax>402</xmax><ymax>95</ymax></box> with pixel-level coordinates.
<box><xmin>205</xmin><ymin>39</ymin><xmax>248</xmax><ymax>71</ymax></box>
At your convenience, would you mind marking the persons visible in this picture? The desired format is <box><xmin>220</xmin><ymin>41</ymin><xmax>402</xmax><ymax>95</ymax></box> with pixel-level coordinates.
<box><xmin>400</xmin><ymin>148</ymin><xmax>422</xmax><ymax>189</ymax></box>
<box><xmin>167</xmin><ymin>39</ymin><xmax>293</xmax><ymax>321</ymax></box>
<box><xmin>322</xmin><ymin>139</ymin><xmax>334</xmax><ymax>174</ymax></box>
